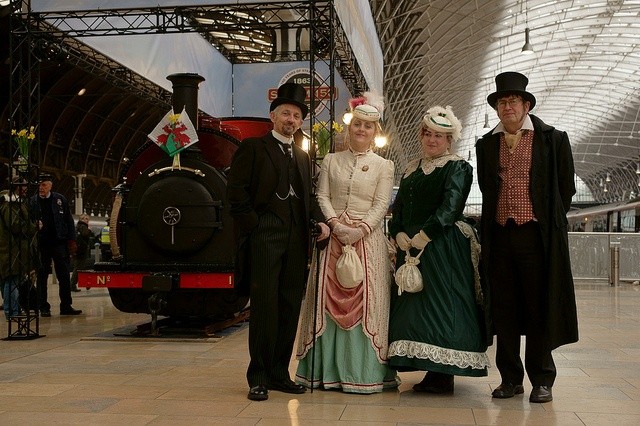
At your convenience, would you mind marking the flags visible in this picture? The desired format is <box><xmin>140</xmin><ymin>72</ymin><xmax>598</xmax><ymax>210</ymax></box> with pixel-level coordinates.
<box><xmin>149</xmin><ymin>110</ymin><xmax>174</xmax><ymax>153</ymax></box>
<box><xmin>166</xmin><ymin>110</ymin><xmax>200</xmax><ymax>154</ymax></box>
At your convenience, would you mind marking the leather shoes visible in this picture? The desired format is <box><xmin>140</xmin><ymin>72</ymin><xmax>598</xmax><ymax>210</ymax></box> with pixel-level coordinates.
<box><xmin>267</xmin><ymin>378</ymin><xmax>305</xmax><ymax>394</ymax></box>
<box><xmin>247</xmin><ymin>385</ymin><xmax>269</xmax><ymax>401</ymax></box>
<box><xmin>529</xmin><ymin>385</ymin><xmax>553</xmax><ymax>403</ymax></box>
<box><xmin>40</xmin><ymin>311</ymin><xmax>51</xmax><ymax>317</ymax></box>
<box><xmin>60</xmin><ymin>303</ymin><xmax>83</xmax><ymax>315</ymax></box>
<box><xmin>491</xmin><ymin>381</ymin><xmax>524</xmax><ymax>399</ymax></box>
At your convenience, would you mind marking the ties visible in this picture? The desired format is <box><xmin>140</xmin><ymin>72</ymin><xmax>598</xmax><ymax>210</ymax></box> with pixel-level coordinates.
<box><xmin>273</xmin><ymin>135</ymin><xmax>294</xmax><ymax>161</ymax></box>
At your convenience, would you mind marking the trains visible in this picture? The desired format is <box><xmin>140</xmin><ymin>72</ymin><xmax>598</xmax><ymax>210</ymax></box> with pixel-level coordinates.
<box><xmin>78</xmin><ymin>73</ymin><xmax>303</xmax><ymax>345</ymax></box>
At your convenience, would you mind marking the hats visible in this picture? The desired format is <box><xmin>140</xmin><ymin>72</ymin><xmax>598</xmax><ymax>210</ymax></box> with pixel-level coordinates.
<box><xmin>349</xmin><ymin>90</ymin><xmax>386</xmax><ymax>122</ymax></box>
<box><xmin>39</xmin><ymin>173</ymin><xmax>52</xmax><ymax>181</ymax></box>
<box><xmin>423</xmin><ymin>105</ymin><xmax>463</xmax><ymax>142</ymax></box>
<box><xmin>487</xmin><ymin>71</ymin><xmax>536</xmax><ymax>112</ymax></box>
<box><xmin>270</xmin><ymin>83</ymin><xmax>308</xmax><ymax>120</ymax></box>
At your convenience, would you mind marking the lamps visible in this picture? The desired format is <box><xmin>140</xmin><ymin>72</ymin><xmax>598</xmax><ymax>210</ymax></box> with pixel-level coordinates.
<box><xmin>606</xmin><ymin>172</ymin><xmax>610</xmax><ymax>181</ymax></box>
<box><xmin>521</xmin><ymin>0</ymin><xmax>534</xmax><ymax>55</ymax></box>
<box><xmin>467</xmin><ymin>141</ymin><xmax>472</xmax><ymax>161</ymax></box>
<box><xmin>636</xmin><ymin>163</ymin><xmax>640</xmax><ymax>174</ymax></box>
<box><xmin>474</xmin><ymin>125</ymin><xmax>478</xmax><ymax>147</ymax></box>
<box><xmin>483</xmin><ymin>103</ymin><xmax>490</xmax><ymax>128</ymax></box>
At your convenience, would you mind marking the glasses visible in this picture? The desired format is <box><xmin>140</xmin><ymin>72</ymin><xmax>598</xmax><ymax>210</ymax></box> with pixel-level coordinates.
<box><xmin>495</xmin><ymin>98</ymin><xmax>524</xmax><ymax>108</ymax></box>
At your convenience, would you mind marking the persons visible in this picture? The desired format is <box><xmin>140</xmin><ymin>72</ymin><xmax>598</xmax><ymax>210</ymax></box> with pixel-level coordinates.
<box><xmin>388</xmin><ymin>105</ymin><xmax>489</xmax><ymax>393</ymax></box>
<box><xmin>228</xmin><ymin>82</ymin><xmax>331</xmax><ymax>403</ymax></box>
<box><xmin>475</xmin><ymin>70</ymin><xmax>579</xmax><ymax>402</ymax></box>
<box><xmin>26</xmin><ymin>175</ymin><xmax>83</xmax><ymax>314</ymax></box>
<box><xmin>0</xmin><ymin>176</ymin><xmax>42</xmax><ymax>322</ymax></box>
<box><xmin>70</xmin><ymin>213</ymin><xmax>94</xmax><ymax>291</ymax></box>
<box><xmin>297</xmin><ymin>91</ymin><xmax>401</xmax><ymax>395</ymax></box>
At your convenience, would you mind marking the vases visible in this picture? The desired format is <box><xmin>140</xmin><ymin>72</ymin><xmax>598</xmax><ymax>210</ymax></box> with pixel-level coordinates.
<box><xmin>19</xmin><ymin>155</ymin><xmax>28</xmax><ymax>172</ymax></box>
<box><xmin>318</xmin><ymin>155</ymin><xmax>325</xmax><ymax>174</ymax></box>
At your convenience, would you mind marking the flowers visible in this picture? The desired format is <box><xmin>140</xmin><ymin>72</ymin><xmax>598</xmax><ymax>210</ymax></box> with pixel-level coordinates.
<box><xmin>312</xmin><ymin>121</ymin><xmax>344</xmax><ymax>177</ymax></box>
<box><xmin>12</xmin><ymin>125</ymin><xmax>36</xmax><ymax>172</ymax></box>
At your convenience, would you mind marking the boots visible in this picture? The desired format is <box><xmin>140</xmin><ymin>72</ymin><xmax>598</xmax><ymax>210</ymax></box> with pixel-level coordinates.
<box><xmin>412</xmin><ymin>370</ymin><xmax>454</xmax><ymax>395</ymax></box>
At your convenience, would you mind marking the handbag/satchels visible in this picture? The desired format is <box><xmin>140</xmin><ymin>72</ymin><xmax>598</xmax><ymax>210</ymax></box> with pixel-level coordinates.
<box><xmin>395</xmin><ymin>249</ymin><xmax>424</xmax><ymax>296</ymax></box>
<box><xmin>335</xmin><ymin>236</ymin><xmax>365</xmax><ymax>288</ymax></box>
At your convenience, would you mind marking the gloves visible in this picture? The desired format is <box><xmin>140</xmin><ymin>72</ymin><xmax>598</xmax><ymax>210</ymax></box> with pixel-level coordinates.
<box><xmin>408</xmin><ymin>229</ymin><xmax>432</xmax><ymax>250</ymax></box>
<box><xmin>396</xmin><ymin>231</ymin><xmax>411</xmax><ymax>250</ymax></box>
<box><xmin>332</xmin><ymin>222</ymin><xmax>353</xmax><ymax>237</ymax></box>
<box><xmin>340</xmin><ymin>227</ymin><xmax>364</xmax><ymax>245</ymax></box>
